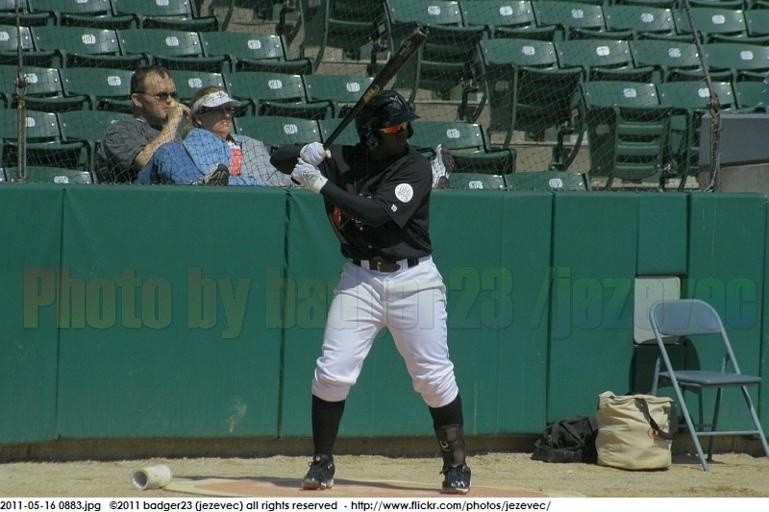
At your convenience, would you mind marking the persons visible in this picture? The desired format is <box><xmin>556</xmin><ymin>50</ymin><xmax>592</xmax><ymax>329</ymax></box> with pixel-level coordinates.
<box><xmin>289</xmin><ymin>88</ymin><xmax>471</xmax><ymax>495</ymax></box>
<box><xmin>184</xmin><ymin>85</ymin><xmax>302</xmax><ymax>187</ymax></box>
<box><xmin>97</xmin><ymin>65</ymin><xmax>230</xmax><ymax>184</ymax></box>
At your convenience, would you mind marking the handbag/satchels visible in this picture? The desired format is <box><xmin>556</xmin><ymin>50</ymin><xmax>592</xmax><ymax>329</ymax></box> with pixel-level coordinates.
<box><xmin>596</xmin><ymin>390</ymin><xmax>673</xmax><ymax>471</ymax></box>
<box><xmin>532</xmin><ymin>415</ymin><xmax>597</xmax><ymax>463</ymax></box>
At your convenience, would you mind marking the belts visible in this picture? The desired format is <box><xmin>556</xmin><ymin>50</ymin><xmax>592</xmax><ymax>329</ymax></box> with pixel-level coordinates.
<box><xmin>352</xmin><ymin>256</ymin><xmax>420</xmax><ymax>272</ymax></box>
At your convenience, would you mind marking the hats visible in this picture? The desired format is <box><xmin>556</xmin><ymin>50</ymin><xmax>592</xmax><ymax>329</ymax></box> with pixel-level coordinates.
<box><xmin>189</xmin><ymin>92</ymin><xmax>244</xmax><ymax>118</ymax></box>
<box><xmin>356</xmin><ymin>89</ymin><xmax>422</xmax><ymax>129</ymax></box>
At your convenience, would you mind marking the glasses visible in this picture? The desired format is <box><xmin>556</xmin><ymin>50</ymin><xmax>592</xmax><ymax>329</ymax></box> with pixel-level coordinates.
<box><xmin>132</xmin><ymin>90</ymin><xmax>178</xmax><ymax>98</ymax></box>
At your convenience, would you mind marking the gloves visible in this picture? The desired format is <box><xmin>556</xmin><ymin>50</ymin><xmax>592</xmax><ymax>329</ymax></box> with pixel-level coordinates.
<box><xmin>291</xmin><ymin>158</ymin><xmax>329</xmax><ymax>194</ymax></box>
<box><xmin>300</xmin><ymin>141</ymin><xmax>332</xmax><ymax>165</ymax></box>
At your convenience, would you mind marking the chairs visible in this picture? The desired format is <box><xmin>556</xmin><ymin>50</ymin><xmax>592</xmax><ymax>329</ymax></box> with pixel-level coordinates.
<box><xmin>0</xmin><ymin>0</ymin><xmax>769</xmax><ymax>191</ymax></box>
<box><xmin>649</xmin><ymin>298</ymin><xmax>769</xmax><ymax>472</ymax></box>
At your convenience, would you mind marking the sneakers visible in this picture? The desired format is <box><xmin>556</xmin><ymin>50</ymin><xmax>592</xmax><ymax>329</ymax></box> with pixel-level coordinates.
<box><xmin>439</xmin><ymin>462</ymin><xmax>471</xmax><ymax>494</ymax></box>
<box><xmin>300</xmin><ymin>454</ymin><xmax>335</xmax><ymax>490</ymax></box>
<box><xmin>189</xmin><ymin>162</ymin><xmax>230</xmax><ymax>187</ymax></box>
